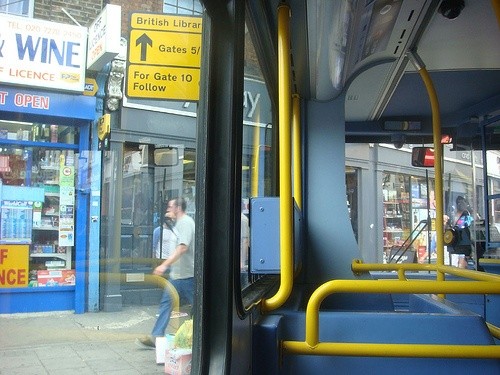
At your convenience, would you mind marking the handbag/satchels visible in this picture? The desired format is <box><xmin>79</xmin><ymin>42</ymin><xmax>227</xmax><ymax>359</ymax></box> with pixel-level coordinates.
<box><xmin>447</xmin><ymin>210</ymin><xmax>472</xmax><ymax>254</ymax></box>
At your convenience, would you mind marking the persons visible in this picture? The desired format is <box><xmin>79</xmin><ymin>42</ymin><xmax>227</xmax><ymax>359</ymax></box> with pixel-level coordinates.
<box><xmin>444</xmin><ymin>196</ymin><xmax>479</xmax><ymax>268</ymax></box>
<box><xmin>139</xmin><ymin>198</ymin><xmax>194</xmax><ymax>348</ymax></box>
<box><xmin>242</xmin><ymin>208</ymin><xmax>250</xmax><ymax>289</ymax></box>
<box><xmin>153</xmin><ymin>220</ymin><xmax>178</xmax><ymax>261</ymax></box>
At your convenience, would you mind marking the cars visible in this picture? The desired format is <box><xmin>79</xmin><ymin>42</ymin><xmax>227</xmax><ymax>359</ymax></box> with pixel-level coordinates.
<box><xmin>77</xmin><ymin>215</ymin><xmax>140</xmax><ymax>258</ymax></box>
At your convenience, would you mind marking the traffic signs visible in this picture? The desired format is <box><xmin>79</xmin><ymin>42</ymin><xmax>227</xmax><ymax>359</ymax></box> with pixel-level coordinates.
<box><xmin>124</xmin><ymin>13</ymin><xmax>203</xmax><ymax>100</ymax></box>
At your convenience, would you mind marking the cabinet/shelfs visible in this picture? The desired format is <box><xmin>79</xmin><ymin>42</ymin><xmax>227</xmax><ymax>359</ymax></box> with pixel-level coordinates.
<box><xmin>0</xmin><ymin>138</ymin><xmax>79</xmax><ymax>293</ymax></box>
<box><xmin>383</xmin><ymin>198</ymin><xmax>411</xmax><ymax>248</ymax></box>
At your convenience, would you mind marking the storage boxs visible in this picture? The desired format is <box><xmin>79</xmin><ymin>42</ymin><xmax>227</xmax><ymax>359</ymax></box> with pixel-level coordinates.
<box><xmin>155</xmin><ymin>336</ymin><xmax>192</xmax><ymax>375</ymax></box>
<box><xmin>36</xmin><ymin>269</ymin><xmax>76</xmax><ymax>285</ymax></box>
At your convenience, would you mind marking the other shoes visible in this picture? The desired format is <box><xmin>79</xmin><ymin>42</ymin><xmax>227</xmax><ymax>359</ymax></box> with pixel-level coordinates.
<box><xmin>138</xmin><ymin>336</ymin><xmax>155</xmax><ymax>347</ymax></box>
